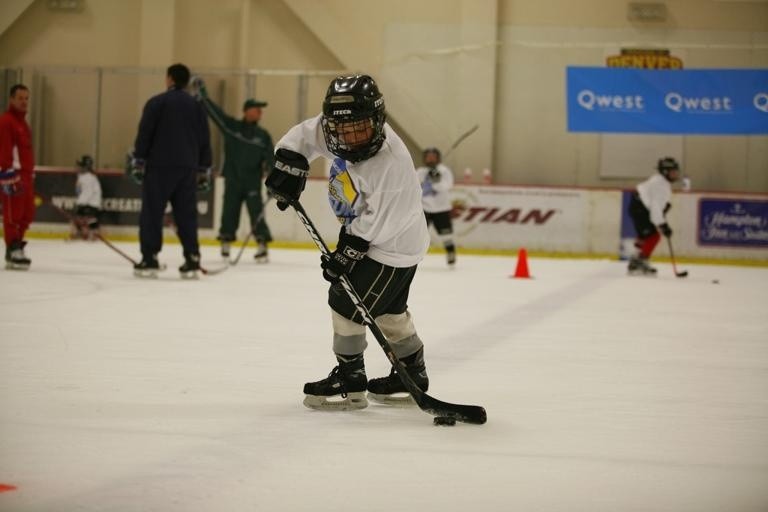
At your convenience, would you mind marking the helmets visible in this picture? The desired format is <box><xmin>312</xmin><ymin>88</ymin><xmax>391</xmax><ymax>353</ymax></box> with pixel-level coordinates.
<box><xmin>422</xmin><ymin>149</ymin><xmax>442</xmax><ymax>166</ymax></box>
<box><xmin>321</xmin><ymin>75</ymin><xmax>385</xmax><ymax>161</ymax></box>
<box><xmin>658</xmin><ymin>158</ymin><xmax>681</xmax><ymax>182</ymax></box>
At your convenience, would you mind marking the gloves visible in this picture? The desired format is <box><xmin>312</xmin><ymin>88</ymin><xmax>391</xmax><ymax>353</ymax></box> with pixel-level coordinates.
<box><xmin>320</xmin><ymin>237</ymin><xmax>372</xmax><ymax>281</ymax></box>
<box><xmin>189</xmin><ymin>76</ymin><xmax>206</xmax><ymax>99</ymax></box>
<box><xmin>265</xmin><ymin>151</ymin><xmax>308</xmax><ymax>211</ymax></box>
<box><xmin>3</xmin><ymin>171</ymin><xmax>20</xmax><ymax>194</ymax></box>
<box><xmin>198</xmin><ymin>172</ymin><xmax>211</xmax><ymax>193</ymax></box>
<box><xmin>131</xmin><ymin>157</ymin><xmax>146</xmax><ymax>185</ymax></box>
<box><xmin>661</xmin><ymin>225</ymin><xmax>671</xmax><ymax>237</ymax></box>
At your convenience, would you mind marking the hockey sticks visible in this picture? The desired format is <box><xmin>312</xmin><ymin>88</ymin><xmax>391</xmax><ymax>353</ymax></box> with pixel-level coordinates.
<box><xmin>229</xmin><ymin>193</ymin><xmax>271</xmax><ymax>267</ymax></box>
<box><xmin>290</xmin><ymin>199</ymin><xmax>488</xmax><ymax>428</ymax></box>
<box><xmin>91</xmin><ymin>231</ymin><xmax>138</xmax><ymax>267</ymax></box>
<box><xmin>434</xmin><ymin>123</ymin><xmax>482</xmax><ymax>169</ymax></box>
<box><xmin>663</xmin><ymin>213</ymin><xmax>689</xmax><ymax>278</ymax></box>
<box><xmin>197</xmin><ymin>265</ymin><xmax>232</xmax><ymax>278</ymax></box>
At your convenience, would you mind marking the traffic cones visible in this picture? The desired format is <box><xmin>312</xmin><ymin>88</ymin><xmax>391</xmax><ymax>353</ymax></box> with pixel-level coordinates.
<box><xmin>516</xmin><ymin>248</ymin><xmax>529</xmax><ymax>277</ymax></box>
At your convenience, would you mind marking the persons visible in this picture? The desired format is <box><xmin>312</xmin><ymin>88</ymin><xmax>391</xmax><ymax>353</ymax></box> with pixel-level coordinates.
<box><xmin>193</xmin><ymin>76</ymin><xmax>273</xmax><ymax>263</ymax></box>
<box><xmin>628</xmin><ymin>159</ymin><xmax>679</xmax><ymax>274</ymax></box>
<box><xmin>1</xmin><ymin>84</ymin><xmax>35</xmax><ymax>270</ymax></box>
<box><xmin>72</xmin><ymin>156</ymin><xmax>103</xmax><ymax>239</ymax></box>
<box><xmin>134</xmin><ymin>64</ymin><xmax>211</xmax><ymax>279</ymax></box>
<box><xmin>415</xmin><ymin>149</ymin><xmax>458</xmax><ymax>265</ymax></box>
<box><xmin>265</xmin><ymin>76</ymin><xmax>430</xmax><ymax>411</ymax></box>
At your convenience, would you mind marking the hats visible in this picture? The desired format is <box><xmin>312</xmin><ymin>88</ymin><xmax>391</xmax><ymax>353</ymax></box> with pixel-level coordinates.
<box><xmin>243</xmin><ymin>99</ymin><xmax>267</xmax><ymax>110</ymax></box>
<box><xmin>77</xmin><ymin>156</ymin><xmax>95</xmax><ymax>174</ymax></box>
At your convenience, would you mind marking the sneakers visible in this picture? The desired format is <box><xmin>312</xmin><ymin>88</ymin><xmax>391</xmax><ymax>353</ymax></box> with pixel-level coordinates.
<box><xmin>254</xmin><ymin>243</ymin><xmax>268</xmax><ymax>259</ymax></box>
<box><xmin>644</xmin><ymin>265</ymin><xmax>656</xmax><ymax>274</ymax></box>
<box><xmin>134</xmin><ymin>253</ymin><xmax>160</xmax><ymax>271</ymax></box>
<box><xmin>303</xmin><ymin>356</ymin><xmax>368</xmax><ymax>396</ymax></box>
<box><xmin>446</xmin><ymin>252</ymin><xmax>456</xmax><ymax>265</ymax></box>
<box><xmin>5</xmin><ymin>246</ymin><xmax>31</xmax><ymax>265</ymax></box>
<box><xmin>628</xmin><ymin>261</ymin><xmax>646</xmax><ymax>274</ymax></box>
<box><xmin>368</xmin><ymin>354</ymin><xmax>432</xmax><ymax>395</ymax></box>
<box><xmin>221</xmin><ymin>241</ymin><xmax>231</xmax><ymax>257</ymax></box>
<box><xmin>179</xmin><ymin>254</ymin><xmax>202</xmax><ymax>272</ymax></box>
